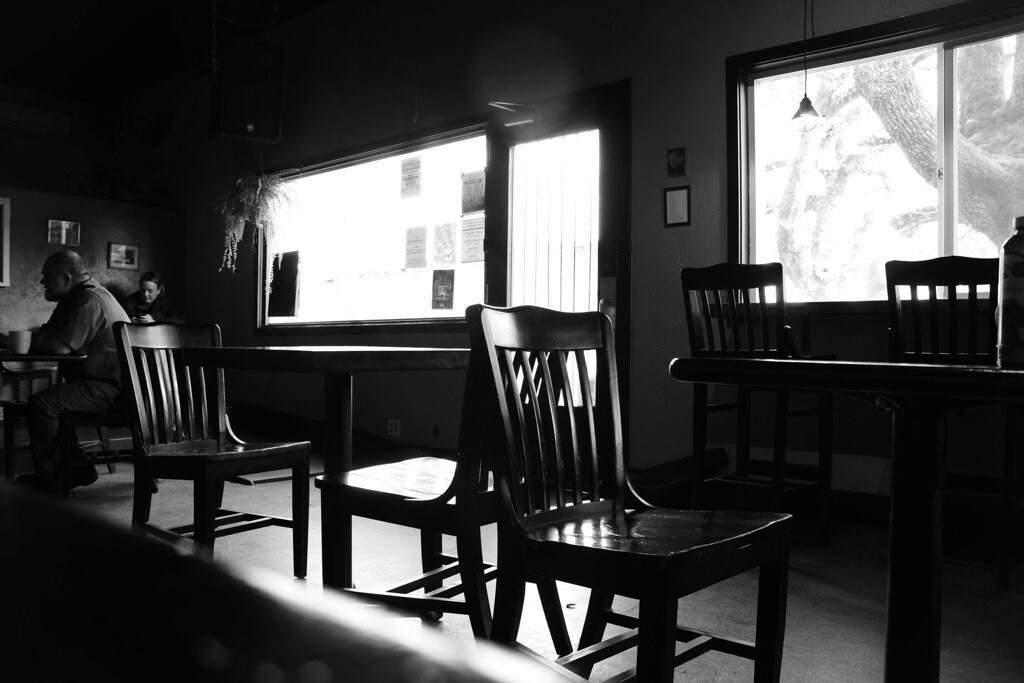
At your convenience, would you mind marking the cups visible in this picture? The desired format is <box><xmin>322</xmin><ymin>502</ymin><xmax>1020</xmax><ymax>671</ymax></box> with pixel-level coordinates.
<box><xmin>8</xmin><ymin>330</ymin><xmax>32</xmax><ymax>354</ymax></box>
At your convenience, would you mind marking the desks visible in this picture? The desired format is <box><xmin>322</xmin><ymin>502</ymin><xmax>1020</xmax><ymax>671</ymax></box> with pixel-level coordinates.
<box><xmin>1</xmin><ymin>352</ymin><xmax>89</xmax><ymax>498</ymax></box>
<box><xmin>666</xmin><ymin>357</ymin><xmax>1023</xmax><ymax>683</ymax></box>
<box><xmin>179</xmin><ymin>343</ymin><xmax>473</xmax><ymax>589</ymax></box>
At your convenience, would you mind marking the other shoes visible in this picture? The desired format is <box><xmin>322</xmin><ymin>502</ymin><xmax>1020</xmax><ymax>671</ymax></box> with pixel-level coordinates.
<box><xmin>17</xmin><ymin>457</ymin><xmax>100</xmax><ymax>492</ymax></box>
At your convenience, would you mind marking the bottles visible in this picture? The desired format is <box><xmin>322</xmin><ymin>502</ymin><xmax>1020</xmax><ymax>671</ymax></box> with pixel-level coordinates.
<box><xmin>1001</xmin><ymin>217</ymin><xmax>1024</xmax><ymax>369</ymax></box>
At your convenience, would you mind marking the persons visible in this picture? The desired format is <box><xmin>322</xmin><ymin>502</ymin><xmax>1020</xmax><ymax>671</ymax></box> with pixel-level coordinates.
<box><xmin>123</xmin><ymin>272</ymin><xmax>186</xmax><ymax>324</ymax></box>
<box><xmin>0</xmin><ymin>250</ymin><xmax>132</xmax><ymax>498</ymax></box>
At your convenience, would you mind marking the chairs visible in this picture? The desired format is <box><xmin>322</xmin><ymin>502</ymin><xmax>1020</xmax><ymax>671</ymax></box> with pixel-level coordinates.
<box><xmin>0</xmin><ymin>362</ymin><xmax>116</xmax><ymax>481</ymax></box>
<box><xmin>682</xmin><ymin>262</ymin><xmax>835</xmax><ymax>549</ymax></box>
<box><xmin>884</xmin><ymin>256</ymin><xmax>1023</xmax><ymax>590</ymax></box>
<box><xmin>111</xmin><ymin>319</ymin><xmax>313</xmax><ymax>581</ymax></box>
<box><xmin>312</xmin><ymin>347</ymin><xmax>574</xmax><ymax>657</ymax></box>
<box><xmin>466</xmin><ymin>304</ymin><xmax>794</xmax><ymax>683</ymax></box>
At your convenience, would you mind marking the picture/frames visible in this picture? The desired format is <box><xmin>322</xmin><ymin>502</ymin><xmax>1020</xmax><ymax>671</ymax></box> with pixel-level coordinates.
<box><xmin>46</xmin><ymin>218</ymin><xmax>81</xmax><ymax>247</ymax></box>
<box><xmin>107</xmin><ymin>241</ymin><xmax>141</xmax><ymax>272</ymax></box>
